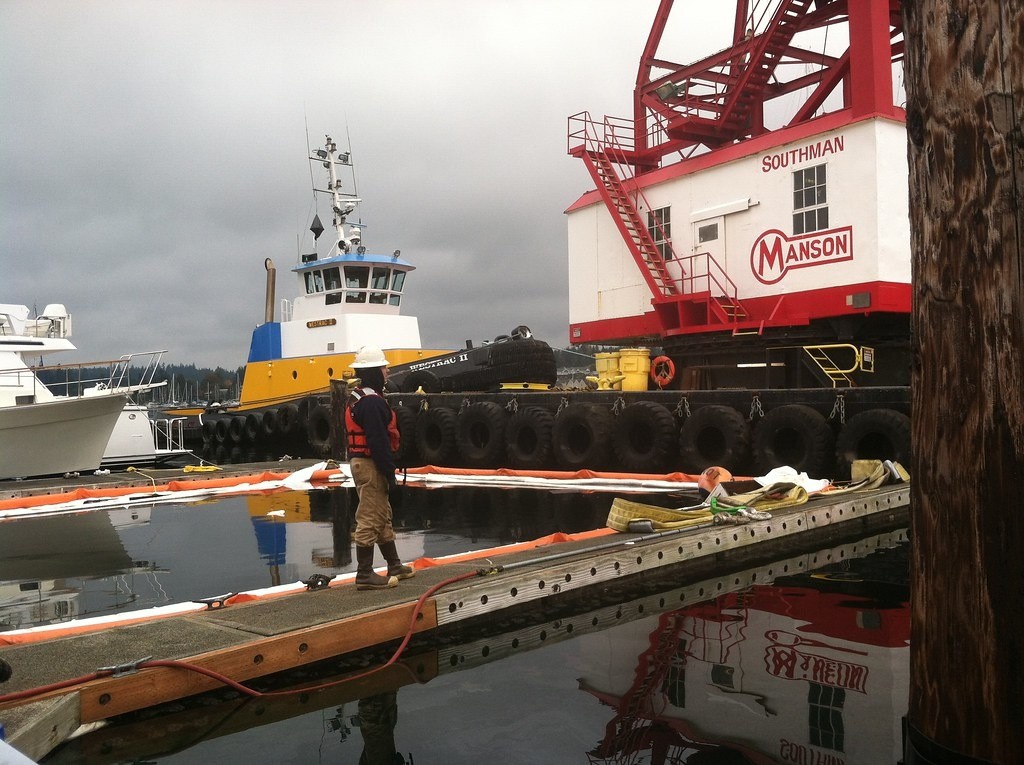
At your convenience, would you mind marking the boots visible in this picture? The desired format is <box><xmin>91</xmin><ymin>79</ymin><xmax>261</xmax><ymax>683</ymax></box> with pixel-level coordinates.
<box><xmin>355</xmin><ymin>545</ymin><xmax>390</xmax><ymax>590</ymax></box>
<box><xmin>378</xmin><ymin>540</ymin><xmax>415</xmax><ymax>580</ymax></box>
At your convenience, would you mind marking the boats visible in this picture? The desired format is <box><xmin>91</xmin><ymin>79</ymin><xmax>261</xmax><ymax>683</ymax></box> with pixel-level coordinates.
<box><xmin>179</xmin><ymin>465</ymin><xmax>224</xmax><ymax>474</ymax></box>
<box><xmin>0</xmin><ymin>300</ymin><xmax>132</xmax><ymax>484</ymax></box>
<box><xmin>151</xmin><ymin>142</ymin><xmax>560</xmax><ymax>439</ymax></box>
<box><xmin>72</xmin><ymin>350</ymin><xmax>194</xmax><ymax>471</ymax></box>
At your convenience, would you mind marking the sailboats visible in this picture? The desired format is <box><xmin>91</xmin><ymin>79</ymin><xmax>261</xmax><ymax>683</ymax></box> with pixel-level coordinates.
<box><xmin>116</xmin><ymin>370</ymin><xmax>242</xmax><ymax>426</ymax></box>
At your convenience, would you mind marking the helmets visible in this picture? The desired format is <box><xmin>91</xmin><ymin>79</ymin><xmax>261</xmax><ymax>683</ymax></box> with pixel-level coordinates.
<box><xmin>348</xmin><ymin>345</ymin><xmax>390</xmax><ymax>368</ymax></box>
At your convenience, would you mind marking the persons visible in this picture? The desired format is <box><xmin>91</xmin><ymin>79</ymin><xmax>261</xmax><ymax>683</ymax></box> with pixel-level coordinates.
<box><xmin>345</xmin><ymin>346</ymin><xmax>416</xmax><ymax>590</ymax></box>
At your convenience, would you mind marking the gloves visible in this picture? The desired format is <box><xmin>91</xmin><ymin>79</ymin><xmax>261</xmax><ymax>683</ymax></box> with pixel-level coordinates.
<box><xmin>384</xmin><ymin>467</ymin><xmax>397</xmax><ymax>494</ymax></box>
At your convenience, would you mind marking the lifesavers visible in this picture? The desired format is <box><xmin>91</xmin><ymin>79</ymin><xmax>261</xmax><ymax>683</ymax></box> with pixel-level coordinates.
<box><xmin>650</xmin><ymin>355</ymin><xmax>674</xmax><ymax>386</ymax></box>
